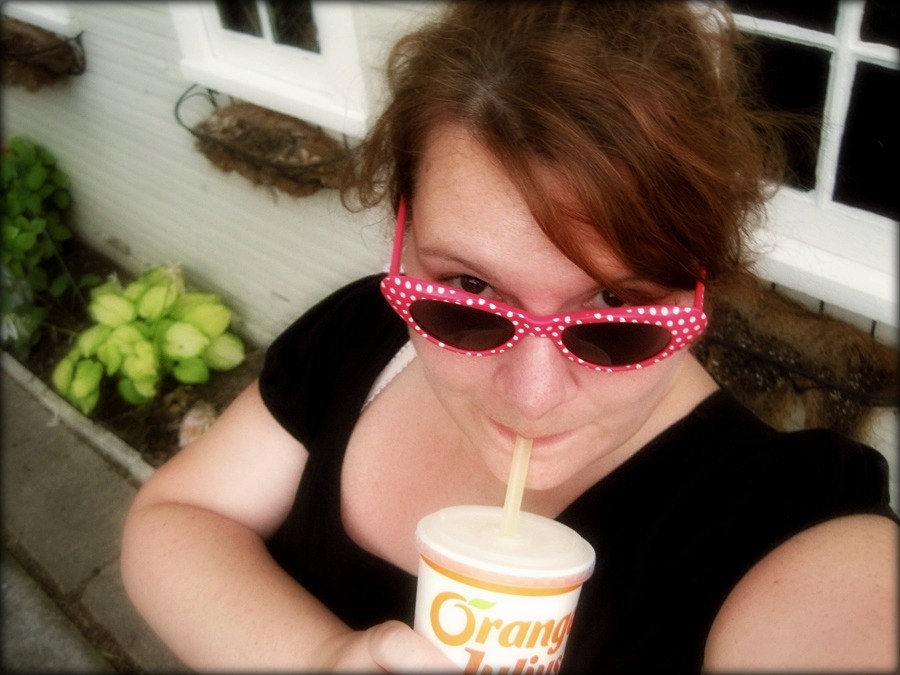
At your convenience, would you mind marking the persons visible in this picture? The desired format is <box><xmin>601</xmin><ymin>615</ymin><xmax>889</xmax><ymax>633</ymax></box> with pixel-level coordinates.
<box><xmin>120</xmin><ymin>1</ymin><xmax>900</xmax><ymax>674</ymax></box>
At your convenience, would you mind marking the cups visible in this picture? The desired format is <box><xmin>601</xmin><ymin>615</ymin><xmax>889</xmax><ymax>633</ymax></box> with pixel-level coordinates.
<box><xmin>410</xmin><ymin>504</ymin><xmax>597</xmax><ymax>675</ymax></box>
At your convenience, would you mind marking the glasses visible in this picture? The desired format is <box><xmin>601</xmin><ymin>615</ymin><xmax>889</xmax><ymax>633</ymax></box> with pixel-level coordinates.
<box><xmin>376</xmin><ymin>197</ymin><xmax>708</xmax><ymax>376</ymax></box>
